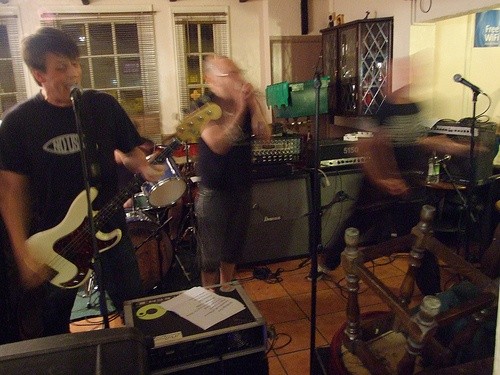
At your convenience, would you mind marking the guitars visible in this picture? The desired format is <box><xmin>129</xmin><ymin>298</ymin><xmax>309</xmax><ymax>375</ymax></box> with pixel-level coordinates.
<box><xmin>26</xmin><ymin>103</ymin><xmax>221</xmax><ymax>290</ymax></box>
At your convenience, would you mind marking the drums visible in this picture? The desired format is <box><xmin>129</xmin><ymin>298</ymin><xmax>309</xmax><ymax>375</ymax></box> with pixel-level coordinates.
<box><xmin>138</xmin><ymin>151</ymin><xmax>189</xmax><ymax>207</ymax></box>
<box><xmin>120</xmin><ymin>207</ymin><xmax>176</xmax><ymax>287</ymax></box>
<box><xmin>132</xmin><ymin>192</ymin><xmax>154</xmax><ymax>212</ymax></box>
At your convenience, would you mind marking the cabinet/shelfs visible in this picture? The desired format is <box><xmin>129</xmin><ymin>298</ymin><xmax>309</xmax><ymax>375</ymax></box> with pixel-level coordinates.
<box><xmin>318</xmin><ymin>16</ymin><xmax>397</xmax><ymax>118</ymax></box>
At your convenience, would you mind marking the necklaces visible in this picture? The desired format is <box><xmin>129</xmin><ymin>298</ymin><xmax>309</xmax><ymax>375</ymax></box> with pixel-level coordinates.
<box><xmin>224</xmin><ymin>107</ymin><xmax>236</xmax><ymax>116</ymax></box>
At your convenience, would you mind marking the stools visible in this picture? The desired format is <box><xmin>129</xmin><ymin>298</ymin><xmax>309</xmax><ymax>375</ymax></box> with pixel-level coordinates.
<box><xmin>330</xmin><ymin>203</ymin><xmax>500</xmax><ymax>375</ymax></box>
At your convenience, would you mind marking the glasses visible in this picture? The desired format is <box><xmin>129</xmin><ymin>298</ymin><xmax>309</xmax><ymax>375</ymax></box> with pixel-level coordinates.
<box><xmin>210</xmin><ymin>69</ymin><xmax>243</xmax><ymax>78</ymax></box>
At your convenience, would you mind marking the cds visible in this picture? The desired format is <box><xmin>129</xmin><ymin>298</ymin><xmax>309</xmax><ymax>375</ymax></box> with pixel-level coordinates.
<box><xmin>137</xmin><ymin>303</ymin><xmax>167</xmax><ymax>321</ymax></box>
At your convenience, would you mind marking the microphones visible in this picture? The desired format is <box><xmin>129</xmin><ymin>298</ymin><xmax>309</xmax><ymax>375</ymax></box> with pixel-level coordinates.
<box><xmin>69</xmin><ymin>86</ymin><xmax>84</xmax><ymax>100</ymax></box>
<box><xmin>436</xmin><ymin>154</ymin><xmax>452</xmax><ymax>164</ymax></box>
<box><xmin>454</xmin><ymin>74</ymin><xmax>488</xmax><ymax>96</ymax></box>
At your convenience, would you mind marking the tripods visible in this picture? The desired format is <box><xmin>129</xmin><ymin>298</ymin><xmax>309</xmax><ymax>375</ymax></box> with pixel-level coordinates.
<box><xmin>175</xmin><ymin>140</ymin><xmax>200</xmax><ymax>271</ymax></box>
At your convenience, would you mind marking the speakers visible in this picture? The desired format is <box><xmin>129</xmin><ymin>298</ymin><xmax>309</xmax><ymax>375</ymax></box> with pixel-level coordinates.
<box><xmin>239</xmin><ymin>173</ymin><xmax>312</xmax><ymax>268</ymax></box>
<box><xmin>317</xmin><ymin>167</ymin><xmax>370</xmax><ymax>251</ymax></box>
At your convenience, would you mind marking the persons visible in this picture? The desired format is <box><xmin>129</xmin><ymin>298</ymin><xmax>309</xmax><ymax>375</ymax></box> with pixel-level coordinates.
<box><xmin>0</xmin><ymin>28</ymin><xmax>277</xmax><ymax>344</ymax></box>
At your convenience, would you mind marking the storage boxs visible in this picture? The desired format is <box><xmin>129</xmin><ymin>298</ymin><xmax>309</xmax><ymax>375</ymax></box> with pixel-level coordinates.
<box><xmin>265</xmin><ymin>76</ymin><xmax>330</xmax><ymax>120</ymax></box>
<box><xmin>127</xmin><ymin>280</ymin><xmax>269</xmax><ymax>375</ymax></box>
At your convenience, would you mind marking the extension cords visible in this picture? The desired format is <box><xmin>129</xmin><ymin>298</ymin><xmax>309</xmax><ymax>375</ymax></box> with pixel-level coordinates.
<box><xmin>307</xmin><ymin>264</ymin><xmax>325</xmax><ymax>281</ymax></box>
